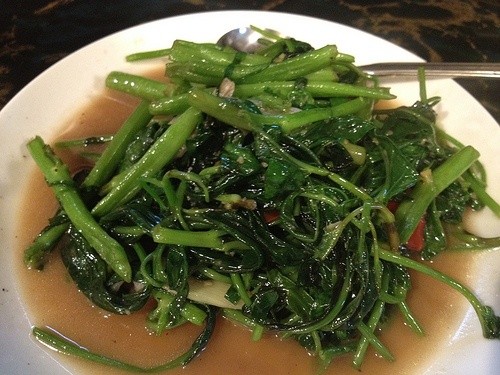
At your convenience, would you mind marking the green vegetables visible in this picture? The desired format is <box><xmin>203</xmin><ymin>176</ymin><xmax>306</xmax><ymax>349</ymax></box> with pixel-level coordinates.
<box><xmin>21</xmin><ymin>24</ymin><xmax>500</xmax><ymax>372</ymax></box>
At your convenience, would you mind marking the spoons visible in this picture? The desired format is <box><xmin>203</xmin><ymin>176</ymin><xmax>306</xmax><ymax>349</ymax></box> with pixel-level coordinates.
<box><xmin>214</xmin><ymin>25</ymin><xmax>500</xmax><ymax>96</ymax></box>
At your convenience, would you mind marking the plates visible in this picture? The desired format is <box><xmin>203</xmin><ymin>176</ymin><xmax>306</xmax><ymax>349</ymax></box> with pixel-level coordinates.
<box><xmin>0</xmin><ymin>8</ymin><xmax>500</xmax><ymax>375</ymax></box>
